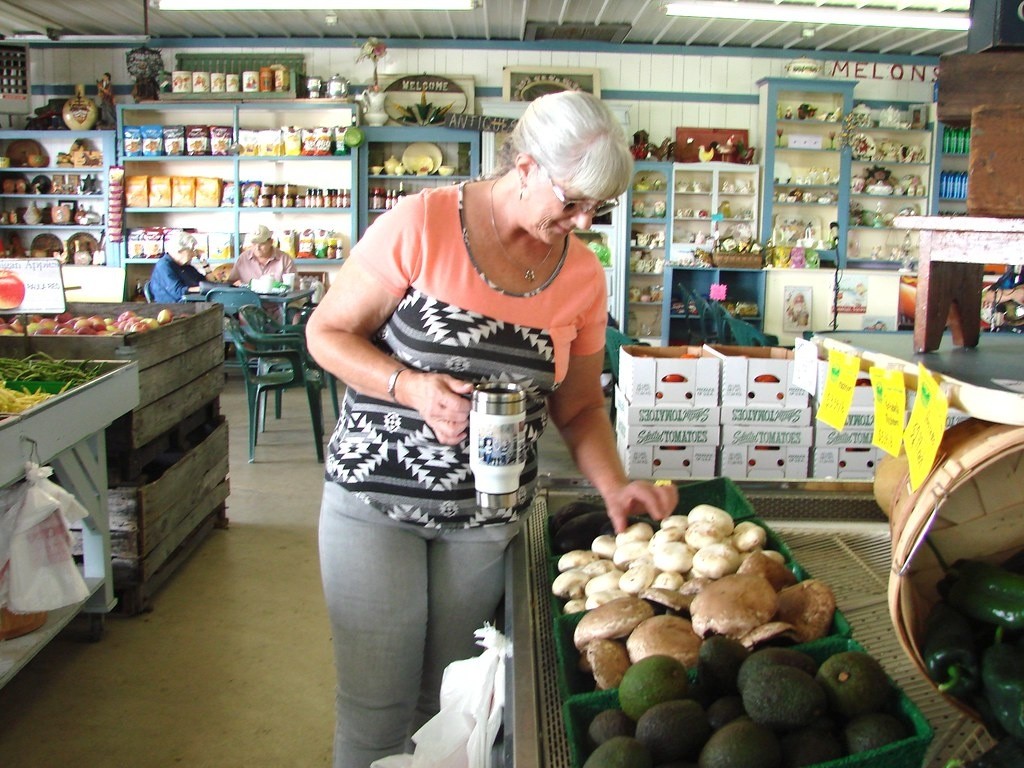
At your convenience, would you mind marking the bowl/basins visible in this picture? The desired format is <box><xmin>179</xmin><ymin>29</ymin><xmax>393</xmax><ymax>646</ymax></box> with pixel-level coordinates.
<box><xmin>439</xmin><ymin>166</ymin><xmax>456</xmax><ymax>176</ymax></box>
<box><xmin>29</xmin><ymin>155</ymin><xmax>49</xmax><ymax>167</ymax></box>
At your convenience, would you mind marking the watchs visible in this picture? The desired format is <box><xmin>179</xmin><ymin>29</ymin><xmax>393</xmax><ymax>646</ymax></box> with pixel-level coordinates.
<box><xmin>388</xmin><ymin>367</ymin><xmax>409</xmax><ymax>404</ymax></box>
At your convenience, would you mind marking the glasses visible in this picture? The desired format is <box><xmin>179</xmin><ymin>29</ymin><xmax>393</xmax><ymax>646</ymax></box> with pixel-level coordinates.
<box><xmin>546</xmin><ymin>168</ymin><xmax>621</xmax><ymax>217</ymax></box>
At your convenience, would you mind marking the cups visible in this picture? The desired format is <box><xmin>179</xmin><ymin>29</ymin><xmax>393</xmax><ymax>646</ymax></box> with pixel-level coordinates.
<box><xmin>52</xmin><ymin>206</ymin><xmax>70</xmax><ymax>225</ymax></box>
<box><xmin>460</xmin><ymin>382</ymin><xmax>529</xmax><ymax>510</ymax></box>
<box><xmin>283</xmin><ymin>273</ymin><xmax>295</xmax><ymax>293</ymax></box>
<box><xmin>172</xmin><ymin>70</ymin><xmax>260</xmax><ymax>93</ymax></box>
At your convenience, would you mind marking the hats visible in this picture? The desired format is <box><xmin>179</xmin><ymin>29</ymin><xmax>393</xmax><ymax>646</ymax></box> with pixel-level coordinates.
<box><xmin>252</xmin><ymin>224</ymin><xmax>273</xmax><ymax>244</ymax></box>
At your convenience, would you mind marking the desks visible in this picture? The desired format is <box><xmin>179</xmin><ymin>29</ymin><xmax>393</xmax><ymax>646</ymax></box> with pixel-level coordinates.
<box><xmin>892</xmin><ymin>214</ymin><xmax>1024</xmax><ymax>351</ymax></box>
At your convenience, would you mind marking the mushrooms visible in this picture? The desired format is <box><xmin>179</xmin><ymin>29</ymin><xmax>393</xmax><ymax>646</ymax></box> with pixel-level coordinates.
<box><xmin>552</xmin><ymin>505</ymin><xmax>837</xmax><ymax>690</ymax></box>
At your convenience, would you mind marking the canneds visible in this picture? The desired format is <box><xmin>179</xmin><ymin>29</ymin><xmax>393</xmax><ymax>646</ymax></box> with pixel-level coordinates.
<box><xmin>258</xmin><ymin>185</ymin><xmax>306</xmax><ymax>208</ymax></box>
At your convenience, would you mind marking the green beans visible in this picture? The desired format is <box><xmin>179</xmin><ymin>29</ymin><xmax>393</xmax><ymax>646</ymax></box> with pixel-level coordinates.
<box><xmin>0</xmin><ymin>350</ymin><xmax>107</xmax><ymax>413</ymax></box>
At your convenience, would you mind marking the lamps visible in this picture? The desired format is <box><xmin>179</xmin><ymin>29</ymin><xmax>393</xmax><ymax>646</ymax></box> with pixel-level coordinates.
<box><xmin>660</xmin><ymin>0</ymin><xmax>971</xmax><ymax>31</ymax></box>
<box><xmin>150</xmin><ymin>0</ymin><xmax>482</xmax><ymax>12</ymax></box>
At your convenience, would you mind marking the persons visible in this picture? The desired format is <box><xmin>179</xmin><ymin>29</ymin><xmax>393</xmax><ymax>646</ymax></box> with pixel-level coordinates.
<box><xmin>96</xmin><ymin>72</ymin><xmax>117</xmax><ymax>131</ymax></box>
<box><xmin>227</xmin><ymin>226</ymin><xmax>302</xmax><ymax>325</ymax></box>
<box><xmin>306</xmin><ymin>87</ymin><xmax>678</xmax><ymax>768</ymax></box>
<box><xmin>151</xmin><ymin>228</ymin><xmax>211</xmax><ymax>304</ymax></box>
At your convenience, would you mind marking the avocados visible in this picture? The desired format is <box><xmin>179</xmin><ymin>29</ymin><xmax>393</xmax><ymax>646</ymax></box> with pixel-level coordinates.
<box><xmin>579</xmin><ymin>634</ymin><xmax>910</xmax><ymax>768</ymax></box>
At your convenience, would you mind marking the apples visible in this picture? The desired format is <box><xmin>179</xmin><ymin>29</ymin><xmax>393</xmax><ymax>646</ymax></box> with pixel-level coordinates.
<box><xmin>0</xmin><ymin>309</ymin><xmax>173</xmax><ymax>336</ymax></box>
<box><xmin>0</xmin><ymin>269</ymin><xmax>26</xmax><ymax>309</ymax></box>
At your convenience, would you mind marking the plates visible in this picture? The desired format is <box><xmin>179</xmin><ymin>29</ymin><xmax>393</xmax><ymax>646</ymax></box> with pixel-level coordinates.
<box><xmin>403</xmin><ymin>143</ymin><xmax>443</xmax><ymax>176</ymax></box>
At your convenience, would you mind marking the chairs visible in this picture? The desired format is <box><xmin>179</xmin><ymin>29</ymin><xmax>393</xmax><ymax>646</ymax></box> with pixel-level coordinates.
<box><xmin>224</xmin><ymin>320</ymin><xmax>327</xmax><ymax>463</ymax></box>
<box><xmin>237</xmin><ymin>300</ymin><xmax>347</xmax><ymax>418</ymax></box>
<box><xmin>205</xmin><ymin>287</ymin><xmax>264</xmax><ymax>332</ymax></box>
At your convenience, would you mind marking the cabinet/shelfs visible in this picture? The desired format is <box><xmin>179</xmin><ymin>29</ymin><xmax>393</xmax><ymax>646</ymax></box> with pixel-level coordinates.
<box><xmin>182</xmin><ymin>288</ymin><xmax>317</xmax><ymax>418</ymax></box>
<box><xmin>0</xmin><ymin>37</ymin><xmax>943</xmax><ymax>350</ymax></box>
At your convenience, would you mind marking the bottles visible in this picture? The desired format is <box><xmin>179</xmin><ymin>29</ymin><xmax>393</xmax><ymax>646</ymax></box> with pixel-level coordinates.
<box><xmin>63</xmin><ymin>83</ymin><xmax>98</xmax><ymax>131</ymax></box>
<box><xmin>299</xmin><ymin>275</ymin><xmax>325</xmax><ymax>304</ymax></box>
<box><xmin>23</xmin><ymin>199</ymin><xmax>43</xmax><ymax>225</ymax></box>
<box><xmin>369</xmin><ymin>182</ymin><xmax>408</xmax><ymax>210</ymax></box>
<box><xmin>9</xmin><ymin>209</ymin><xmax>18</xmax><ymax>224</ymax></box>
<box><xmin>261</xmin><ymin>64</ymin><xmax>290</xmax><ymax>93</ymax></box>
<box><xmin>939</xmin><ymin>125</ymin><xmax>971</xmax><ymax>198</ymax></box>
<box><xmin>258</xmin><ymin>183</ymin><xmax>351</xmax><ymax>208</ymax></box>
<box><xmin>75</xmin><ymin>204</ymin><xmax>100</xmax><ymax>224</ymax></box>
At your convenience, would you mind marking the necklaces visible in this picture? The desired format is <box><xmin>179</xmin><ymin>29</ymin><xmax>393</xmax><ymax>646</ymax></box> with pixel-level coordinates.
<box><xmin>491</xmin><ymin>179</ymin><xmax>556</xmax><ymax>283</ymax></box>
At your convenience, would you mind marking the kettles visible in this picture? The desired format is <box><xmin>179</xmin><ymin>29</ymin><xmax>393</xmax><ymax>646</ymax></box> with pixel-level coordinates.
<box><xmin>301</xmin><ymin>73</ymin><xmax>351</xmax><ymax>99</ymax></box>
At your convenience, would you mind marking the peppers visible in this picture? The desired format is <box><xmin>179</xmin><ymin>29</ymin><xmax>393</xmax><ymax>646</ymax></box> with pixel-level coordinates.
<box><xmin>924</xmin><ymin>548</ymin><xmax>1024</xmax><ymax>768</ymax></box>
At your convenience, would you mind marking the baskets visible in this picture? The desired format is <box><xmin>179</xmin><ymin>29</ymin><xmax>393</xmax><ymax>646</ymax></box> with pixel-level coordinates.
<box><xmin>546</xmin><ymin>477</ymin><xmax>937</xmax><ymax>768</ymax></box>
<box><xmin>712</xmin><ymin>251</ymin><xmax>763</xmax><ymax>270</ymax></box>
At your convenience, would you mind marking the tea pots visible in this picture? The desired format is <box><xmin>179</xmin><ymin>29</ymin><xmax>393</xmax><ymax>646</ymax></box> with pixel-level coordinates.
<box><xmin>370</xmin><ymin>154</ymin><xmax>406</xmax><ymax>176</ymax></box>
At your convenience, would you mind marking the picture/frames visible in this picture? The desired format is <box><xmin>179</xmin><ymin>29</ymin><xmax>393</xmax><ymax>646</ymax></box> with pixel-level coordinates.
<box><xmin>59</xmin><ymin>201</ymin><xmax>77</xmax><ymax>221</ymax></box>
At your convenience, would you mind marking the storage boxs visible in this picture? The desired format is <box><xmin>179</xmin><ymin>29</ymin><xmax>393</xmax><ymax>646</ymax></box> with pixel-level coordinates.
<box><xmin>615</xmin><ymin>343</ymin><xmax>972</xmax><ymax>483</ymax></box>
<box><xmin>0</xmin><ymin>303</ymin><xmax>231</xmax><ymax>613</ymax></box>
